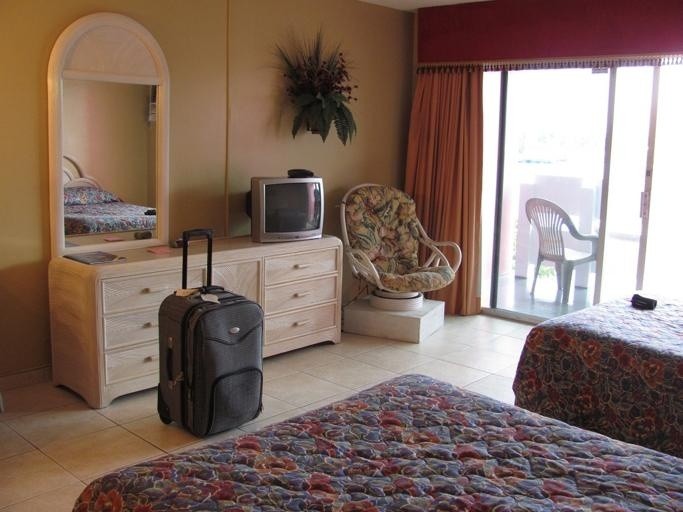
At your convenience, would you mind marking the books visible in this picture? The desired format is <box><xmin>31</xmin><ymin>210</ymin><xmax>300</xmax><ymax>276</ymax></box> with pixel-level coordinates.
<box><xmin>63</xmin><ymin>251</ymin><xmax>127</xmax><ymax>265</ymax></box>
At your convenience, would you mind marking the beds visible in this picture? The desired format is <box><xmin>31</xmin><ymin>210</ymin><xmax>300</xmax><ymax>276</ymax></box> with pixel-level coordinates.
<box><xmin>512</xmin><ymin>298</ymin><xmax>683</xmax><ymax>457</ymax></box>
<box><xmin>72</xmin><ymin>374</ymin><xmax>682</xmax><ymax>512</ymax></box>
<box><xmin>61</xmin><ymin>152</ymin><xmax>156</xmax><ymax>236</ymax></box>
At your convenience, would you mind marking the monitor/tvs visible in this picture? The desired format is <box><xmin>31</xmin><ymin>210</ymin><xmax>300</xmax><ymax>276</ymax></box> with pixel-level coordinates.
<box><xmin>245</xmin><ymin>177</ymin><xmax>325</xmax><ymax>243</ymax></box>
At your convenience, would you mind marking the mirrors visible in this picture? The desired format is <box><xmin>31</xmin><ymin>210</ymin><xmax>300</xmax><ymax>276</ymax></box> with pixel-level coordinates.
<box><xmin>46</xmin><ymin>12</ymin><xmax>171</xmax><ymax>256</ymax></box>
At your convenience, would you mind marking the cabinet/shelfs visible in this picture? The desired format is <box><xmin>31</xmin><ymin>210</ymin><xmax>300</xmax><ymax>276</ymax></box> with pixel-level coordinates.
<box><xmin>48</xmin><ymin>236</ymin><xmax>342</xmax><ymax>408</ymax></box>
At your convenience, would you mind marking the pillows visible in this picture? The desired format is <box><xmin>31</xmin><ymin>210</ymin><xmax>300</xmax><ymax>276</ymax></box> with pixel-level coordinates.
<box><xmin>63</xmin><ymin>187</ymin><xmax>122</xmax><ymax>205</ymax></box>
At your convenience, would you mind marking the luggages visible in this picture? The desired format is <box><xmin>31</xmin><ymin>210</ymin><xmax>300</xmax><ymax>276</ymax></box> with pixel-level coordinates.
<box><xmin>157</xmin><ymin>285</ymin><xmax>265</xmax><ymax>439</ymax></box>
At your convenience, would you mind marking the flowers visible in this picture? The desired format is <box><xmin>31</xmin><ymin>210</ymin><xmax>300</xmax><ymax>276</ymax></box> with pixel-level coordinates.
<box><xmin>274</xmin><ymin>26</ymin><xmax>359</xmax><ymax>146</ymax></box>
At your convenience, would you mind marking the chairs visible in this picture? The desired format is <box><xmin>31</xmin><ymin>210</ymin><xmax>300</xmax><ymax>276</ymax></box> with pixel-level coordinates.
<box><xmin>524</xmin><ymin>197</ymin><xmax>599</xmax><ymax>305</ymax></box>
<box><xmin>340</xmin><ymin>185</ymin><xmax>462</xmax><ymax>344</ymax></box>
